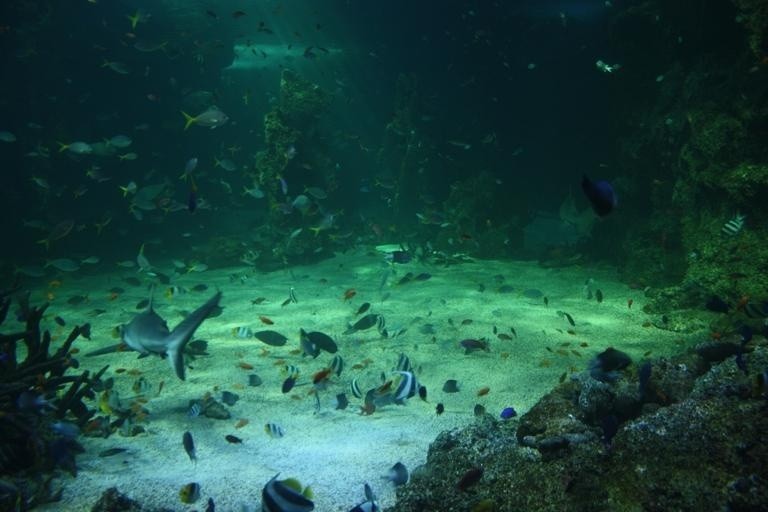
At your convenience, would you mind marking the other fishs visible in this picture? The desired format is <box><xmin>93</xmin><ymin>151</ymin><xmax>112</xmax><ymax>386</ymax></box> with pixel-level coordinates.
<box><xmin>0</xmin><ymin>1</ymin><xmax>767</xmax><ymax>512</ymax></box>
